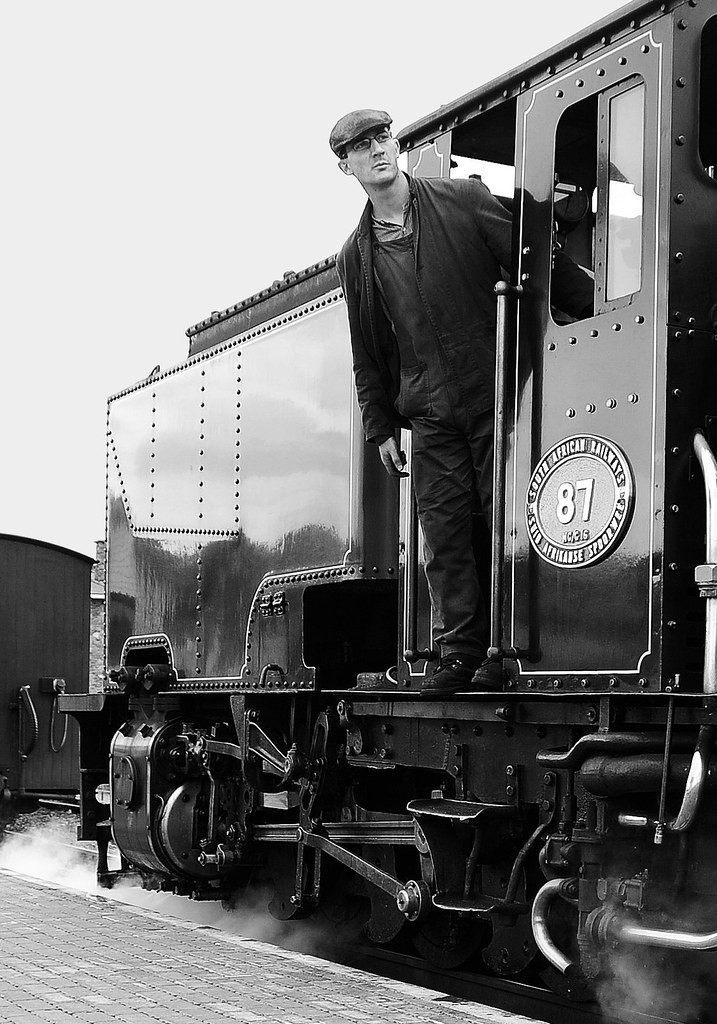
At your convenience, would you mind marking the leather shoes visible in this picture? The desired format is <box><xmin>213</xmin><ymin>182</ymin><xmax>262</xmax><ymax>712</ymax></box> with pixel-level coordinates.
<box><xmin>471</xmin><ymin>658</ymin><xmax>502</xmax><ymax>689</ymax></box>
<box><xmin>421</xmin><ymin>662</ymin><xmax>470</xmax><ymax>695</ymax></box>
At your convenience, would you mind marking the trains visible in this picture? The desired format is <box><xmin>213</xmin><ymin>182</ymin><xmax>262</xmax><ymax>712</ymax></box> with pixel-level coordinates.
<box><xmin>57</xmin><ymin>0</ymin><xmax>717</xmax><ymax>1017</ymax></box>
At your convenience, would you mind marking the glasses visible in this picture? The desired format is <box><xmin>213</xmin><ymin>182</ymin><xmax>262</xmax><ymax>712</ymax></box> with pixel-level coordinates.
<box><xmin>342</xmin><ymin>131</ymin><xmax>392</xmax><ymax>158</ymax></box>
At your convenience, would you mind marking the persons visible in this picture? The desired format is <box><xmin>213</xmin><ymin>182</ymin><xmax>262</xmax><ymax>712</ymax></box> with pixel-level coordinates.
<box><xmin>330</xmin><ymin>108</ymin><xmax>595</xmax><ymax>694</ymax></box>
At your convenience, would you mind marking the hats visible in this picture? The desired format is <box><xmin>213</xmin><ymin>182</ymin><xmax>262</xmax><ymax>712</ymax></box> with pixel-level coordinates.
<box><xmin>329</xmin><ymin>109</ymin><xmax>393</xmax><ymax>159</ymax></box>
